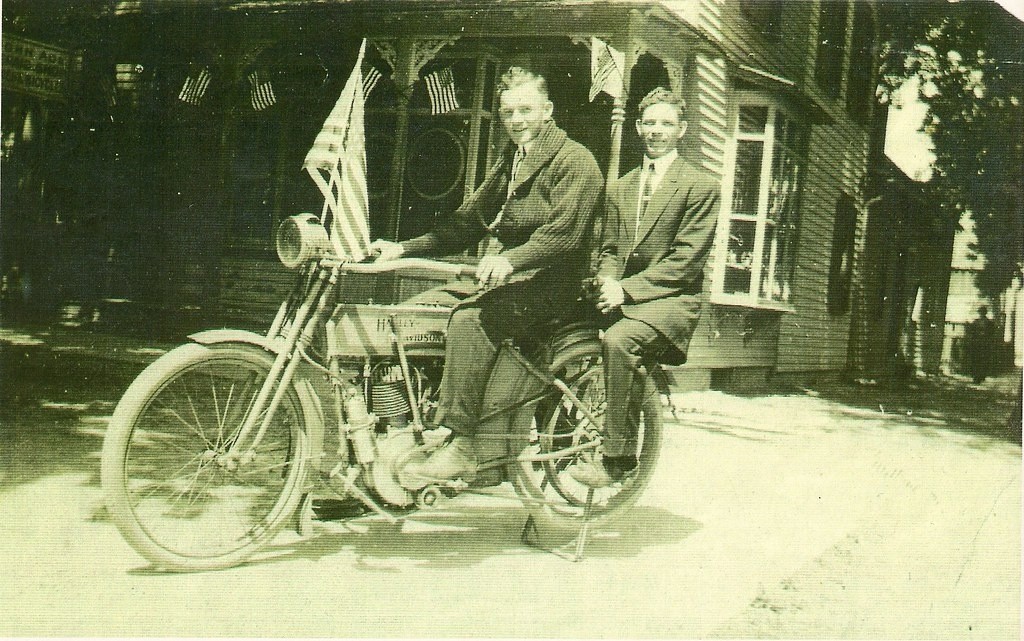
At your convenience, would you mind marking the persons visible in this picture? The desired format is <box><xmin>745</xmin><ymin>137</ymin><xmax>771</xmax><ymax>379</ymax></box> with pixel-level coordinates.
<box><xmin>370</xmin><ymin>66</ymin><xmax>605</xmax><ymax>484</ymax></box>
<box><xmin>534</xmin><ymin>86</ymin><xmax>722</xmax><ymax>488</ymax></box>
<box><xmin>966</xmin><ymin>306</ymin><xmax>994</xmax><ymax>384</ymax></box>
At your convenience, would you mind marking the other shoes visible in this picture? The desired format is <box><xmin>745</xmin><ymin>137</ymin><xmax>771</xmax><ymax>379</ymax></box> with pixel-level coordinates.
<box><xmin>403</xmin><ymin>432</ymin><xmax>478</xmax><ymax>483</ymax></box>
<box><xmin>529</xmin><ymin>449</ymin><xmax>570</xmax><ymax>474</ymax></box>
<box><xmin>566</xmin><ymin>458</ymin><xmax>640</xmax><ymax>487</ymax></box>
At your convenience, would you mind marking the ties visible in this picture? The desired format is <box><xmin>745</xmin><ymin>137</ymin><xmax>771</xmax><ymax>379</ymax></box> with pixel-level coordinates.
<box><xmin>638</xmin><ymin>163</ymin><xmax>654</xmax><ymax>234</ymax></box>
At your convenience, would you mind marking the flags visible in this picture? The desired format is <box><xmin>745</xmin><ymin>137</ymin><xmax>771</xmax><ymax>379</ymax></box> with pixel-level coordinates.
<box><xmin>177</xmin><ymin>62</ymin><xmax>214</xmax><ymax>106</ymax></box>
<box><xmin>301</xmin><ymin>60</ymin><xmax>373</xmax><ymax>264</ymax></box>
<box><xmin>247</xmin><ymin>66</ymin><xmax>277</xmax><ymax>112</ymax></box>
<box><xmin>589</xmin><ymin>47</ymin><xmax>617</xmax><ymax>102</ymax></box>
<box><xmin>424</xmin><ymin>66</ymin><xmax>460</xmax><ymax>115</ymax></box>
<box><xmin>361</xmin><ymin>61</ymin><xmax>382</xmax><ymax>103</ymax></box>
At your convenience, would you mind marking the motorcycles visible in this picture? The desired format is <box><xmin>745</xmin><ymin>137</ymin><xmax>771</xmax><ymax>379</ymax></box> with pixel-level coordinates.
<box><xmin>101</xmin><ymin>210</ymin><xmax>664</xmax><ymax>575</ymax></box>
